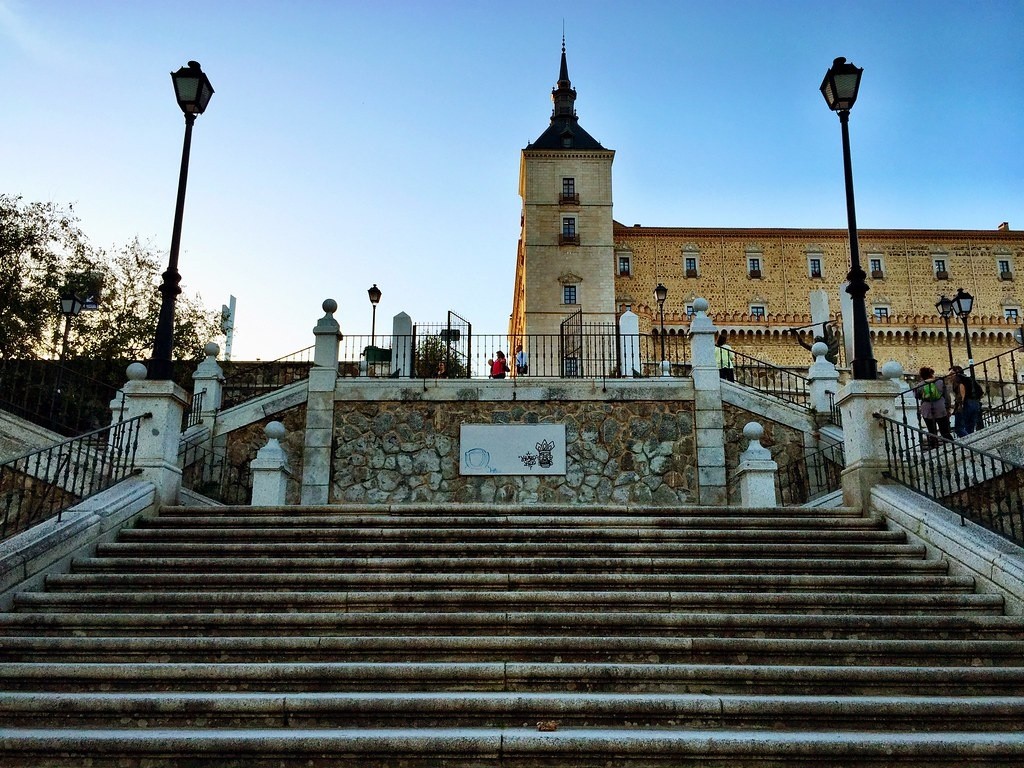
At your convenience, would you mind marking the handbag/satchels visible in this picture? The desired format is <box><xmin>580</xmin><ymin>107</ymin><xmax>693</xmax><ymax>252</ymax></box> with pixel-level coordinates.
<box><xmin>503</xmin><ymin>364</ymin><xmax>510</xmax><ymax>372</ymax></box>
<box><xmin>517</xmin><ymin>364</ymin><xmax>527</xmax><ymax>374</ymax></box>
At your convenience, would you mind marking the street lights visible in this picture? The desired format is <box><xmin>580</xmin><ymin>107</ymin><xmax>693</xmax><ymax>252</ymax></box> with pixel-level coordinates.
<box><xmin>934</xmin><ymin>287</ymin><xmax>975</xmax><ymax>381</ymax></box>
<box><xmin>146</xmin><ymin>61</ymin><xmax>214</xmax><ymax>382</ymax></box>
<box><xmin>653</xmin><ymin>284</ymin><xmax>668</xmax><ymax>360</ymax></box>
<box><xmin>819</xmin><ymin>56</ymin><xmax>877</xmax><ymax>379</ymax></box>
<box><xmin>51</xmin><ymin>289</ymin><xmax>82</xmax><ymax>433</ymax></box>
<box><xmin>368</xmin><ymin>284</ymin><xmax>382</xmax><ymax>347</ymax></box>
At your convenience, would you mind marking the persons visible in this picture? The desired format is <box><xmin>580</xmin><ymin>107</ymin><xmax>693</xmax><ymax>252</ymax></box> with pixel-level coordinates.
<box><xmin>715</xmin><ymin>335</ymin><xmax>735</xmax><ymax>382</ymax></box>
<box><xmin>488</xmin><ymin>351</ymin><xmax>506</xmax><ymax>379</ymax></box>
<box><xmin>949</xmin><ymin>366</ymin><xmax>985</xmax><ymax>438</ymax></box>
<box><xmin>515</xmin><ymin>345</ymin><xmax>527</xmax><ymax>377</ymax></box>
<box><xmin>435</xmin><ymin>361</ymin><xmax>449</xmax><ymax>379</ymax></box>
<box><xmin>915</xmin><ymin>367</ymin><xmax>954</xmax><ymax>448</ymax></box>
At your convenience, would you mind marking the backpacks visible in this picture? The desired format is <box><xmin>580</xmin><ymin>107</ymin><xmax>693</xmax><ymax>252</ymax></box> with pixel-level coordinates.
<box><xmin>967</xmin><ymin>376</ymin><xmax>983</xmax><ymax>400</ymax></box>
<box><xmin>921</xmin><ymin>380</ymin><xmax>943</xmax><ymax>404</ymax></box>
